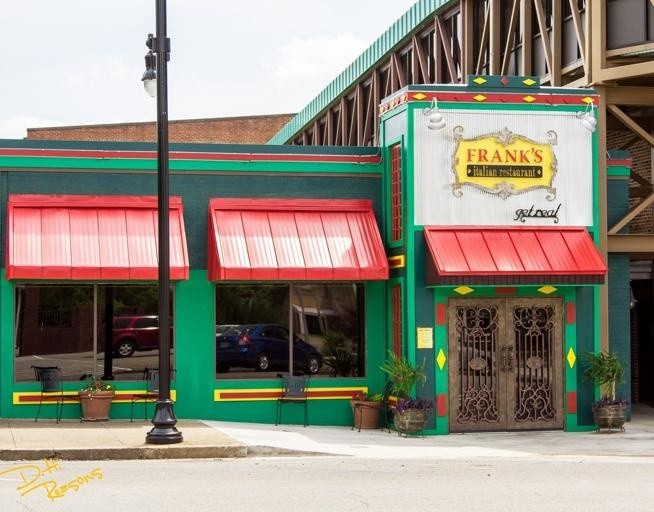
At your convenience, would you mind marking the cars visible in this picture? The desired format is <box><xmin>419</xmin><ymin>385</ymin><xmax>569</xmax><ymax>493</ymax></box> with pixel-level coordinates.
<box><xmin>216</xmin><ymin>324</ymin><xmax>323</xmax><ymax>376</ymax></box>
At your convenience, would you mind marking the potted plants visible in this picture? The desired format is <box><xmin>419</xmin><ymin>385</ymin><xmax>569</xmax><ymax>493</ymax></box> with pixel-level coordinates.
<box><xmin>378</xmin><ymin>349</ymin><xmax>434</xmax><ymax>438</ymax></box>
<box><xmin>579</xmin><ymin>349</ymin><xmax>629</xmax><ymax>434</ymax></box>
<box><xmin>349</xmin><ymin>392</ymin><xmax>383</xmax><ymax>429</ymax></box>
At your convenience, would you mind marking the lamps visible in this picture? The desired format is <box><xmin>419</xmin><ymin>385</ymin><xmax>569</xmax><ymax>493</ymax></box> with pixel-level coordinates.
<box><xmin>576</xmin><ymin>101</ymin><xmax>597</xmax><ymax>133</ymax></box>
<box><xmin>423</xmin><ymin>96</ymin><xmax>447</xmax><ymax>131</ymax></box>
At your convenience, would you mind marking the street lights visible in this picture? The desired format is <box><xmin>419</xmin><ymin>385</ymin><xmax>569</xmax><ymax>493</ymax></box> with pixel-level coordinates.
<box><xmin>137</xmin><ymin>1</ymin><xmax>187</xmax><ymax>444</ymax></box>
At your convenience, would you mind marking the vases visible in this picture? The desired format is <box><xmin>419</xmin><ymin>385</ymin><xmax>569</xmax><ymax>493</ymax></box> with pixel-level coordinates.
<box><xmin>78</xmin><ymin>391</ymin><xmax>115</xmax><ymax>421</ymax></box>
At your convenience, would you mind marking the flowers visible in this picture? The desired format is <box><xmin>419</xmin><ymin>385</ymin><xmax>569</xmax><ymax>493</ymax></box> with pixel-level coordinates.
<box><xmin>79</xmin><ymin>375</ymin><xmax>117</xmax><ymax>400</ymax></box>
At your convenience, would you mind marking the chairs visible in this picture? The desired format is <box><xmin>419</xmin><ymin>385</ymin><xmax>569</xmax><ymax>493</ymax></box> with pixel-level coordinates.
<box><xmin>275</xmin><ymin>374</ymin><xmax>310</xmax><ymax>427</ymax></box>
<box><xmin>351</xmin><ymin>376</ymin><xmax>397</xmax><ymax>433</ymax></box>
<box><xmin>34</xmin><ymin>367</ymin><xmax>85</xmax><ymax>424</ymax></box>
<box><xmin>30</xmin><ymin>365</ymin><xmax>60</xmax><ymax>381</ymax></box>
<box><xmin>142</xmin><ymin>367</ymin><xmax>159</xmax><ymax>380</ymax></box>
<box><xmin>130</xmin><ymin>368</ymin><xmax>176</xmax><ymax>422</ymax></box>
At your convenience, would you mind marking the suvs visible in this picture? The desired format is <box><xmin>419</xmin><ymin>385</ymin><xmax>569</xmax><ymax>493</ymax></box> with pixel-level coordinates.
<box><xmin>97</xmin><ymin>313</ymin><xmax>173</xmax><ymax>357</ymax></box>
